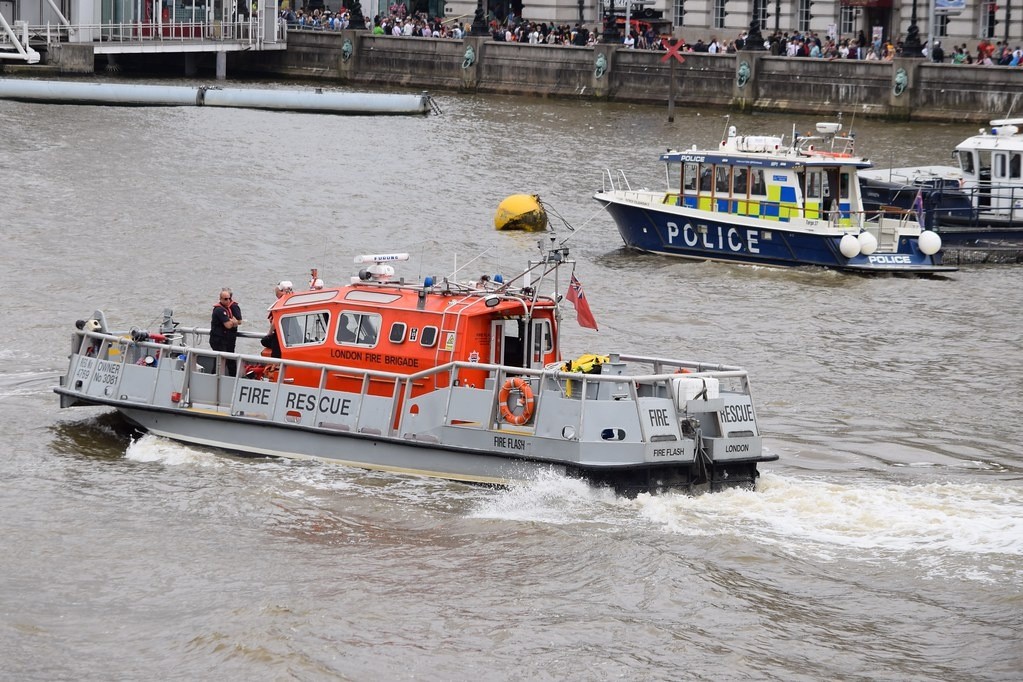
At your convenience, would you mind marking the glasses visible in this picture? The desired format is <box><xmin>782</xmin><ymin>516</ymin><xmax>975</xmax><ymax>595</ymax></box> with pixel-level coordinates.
<box><xmin>221</xmin><ymin>298</ymin><xmax>231</xmax><ymax>301</ymax></box>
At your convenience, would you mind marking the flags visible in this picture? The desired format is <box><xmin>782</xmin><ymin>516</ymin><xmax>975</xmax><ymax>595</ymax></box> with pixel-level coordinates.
<box><xmin>565</xmin><ymin>275</ymin><xmax>598</xmax><ymax>331</ymax></box>
<box><xmin>913</xmin><ymin>191</ymin><xmax>923</xmax><ymax>225</ymax></box>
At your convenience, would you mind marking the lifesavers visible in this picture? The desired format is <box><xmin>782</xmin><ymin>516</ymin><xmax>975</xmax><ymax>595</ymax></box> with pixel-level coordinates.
<box><xmin>498</xmin><ymin>378</ymin><xmax>535</xmax><ymax>426</ymax></box>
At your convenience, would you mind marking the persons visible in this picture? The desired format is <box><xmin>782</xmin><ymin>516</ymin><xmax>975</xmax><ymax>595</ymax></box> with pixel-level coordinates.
<box><xmin>278</xmin><ymin>5</ymin><xmax>350</xmax><ymax>31</ymax></box>
<box><xmin>222</xmin><ymin>289</ymin><xmax>242</xmax><ymax>326</ymax></box>
<box><xmin>623</xmin><ymin>30</ymin><xmax>746</xmax><ymax>53</ymax></box>
<box><xmin>490</xmin><ymin>9</ymin><xmax>600</xmax><ymax>46</ymax></box>
<box><xmin>209</xmin><ymin>292</ymin><xmax>236</xmax><ymax>376</ymax></box>
<box><xmin>736</xmin><ymin>169</ymin><xmax>756</xmax><ymax>193</ymax></box>
<box><xmin>764</xmin><ymin>29</ymin><xmax>902</xmax><ymax>60</ymax></box>
<box><xmin>262</xmin><ymin>281</ymin><xmax>293</xmax><ymax>359</ymax></box>
<box><xmin>364</xmin><ymin>3</ymin><xmax>468</xmax><ymax>39</ymax></box>
<box><xmin>337</xmin><ymin>315</ymin><xmax>361</xmax><ymax>342</ymax></box>
<box><xmin>921</xmin><ymin>38</ymin><xmax>1023</xmax><ymax>67</ymax></box>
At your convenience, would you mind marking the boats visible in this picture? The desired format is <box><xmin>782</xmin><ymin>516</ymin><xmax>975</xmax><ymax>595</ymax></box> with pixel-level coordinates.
<box><xmin>593</xmin><ymin>116</ymin><xmax>960</xmax><ymax>274</ymax></box>
<box><xmin>857</xmin><ymin>118</ymin><xmax>1023</xmax><ymax>241</ymax></box>
<box><xmin>52</xmin><ymin>200</ymin><xmax>781</xmax><ymax>498</ymax></box>
